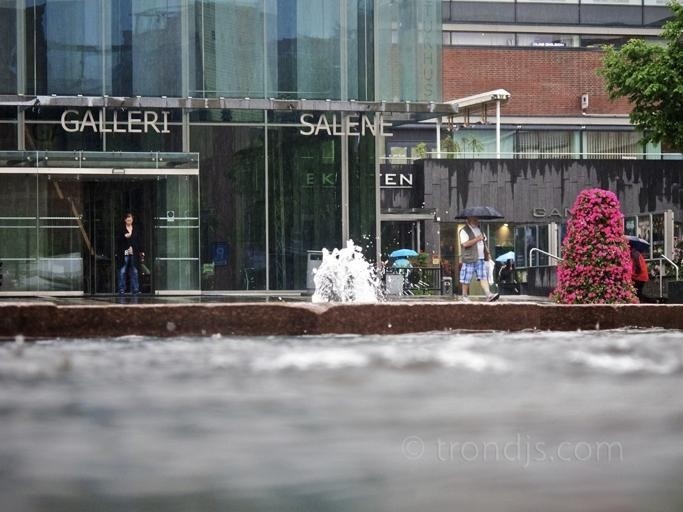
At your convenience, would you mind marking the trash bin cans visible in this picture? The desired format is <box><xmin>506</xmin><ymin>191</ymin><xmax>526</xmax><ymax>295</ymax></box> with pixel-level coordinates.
<box><xmin>306</xmin><ymin>250</ymin><xmax>331</xmax><ymax>289</ymax></box>
<box><xmin>667</xmin><ymin>281</ymin><xmax>683</xmax><ymax>304</ymax></box>
<box><xmin>443</xmin><ymin>278</ymin><xmax>452</xmax><ymax>294</ymax></box>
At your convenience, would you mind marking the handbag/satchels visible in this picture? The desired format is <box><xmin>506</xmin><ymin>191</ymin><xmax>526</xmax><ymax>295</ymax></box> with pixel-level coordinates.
<box><xmin>139</xmin><ymin>251</ymin><xmax>145</xmax><ymax>263</ymax></box>
<box><xmin>481</xmin><ymin>229</ymin><xmax>489</xmax><ymax>261</ymax></box>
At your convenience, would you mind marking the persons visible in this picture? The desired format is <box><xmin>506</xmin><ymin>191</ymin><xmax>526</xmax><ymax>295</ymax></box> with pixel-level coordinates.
<box><xmin>493</xmin><ymin>259</ymin><xmax>520</xmax><ymax>295</ymax></box>
<box><xmin>459</xmin><ymin>216</ymin><xmax>499</xmax><ymax>302</ymax></box>
<box><xmin>118</xmin><ymin>212</ymin><xmax>145</xmax><ymax>295</ymax></box>
<box><xmin>630</xmin><ymin>243</ymin><xmax>659</xmax><ymax>303</ymax></box>
<box><xmin>392</xmin><ymin>257</ymin><xmax>413</xmax><ymax>268</ymax></box>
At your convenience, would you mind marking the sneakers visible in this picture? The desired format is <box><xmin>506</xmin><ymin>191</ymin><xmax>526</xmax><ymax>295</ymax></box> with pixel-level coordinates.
<box><xmin>487</xmin><ymin>293</ymin><xmax>500</xmax><ymax>302</ymax></box>
<box><xmin>462</xmin><ymin>296</ymin><xmax>471</xmax><ymax>302</ymax></box>
<box><xmin>118</xmin><ymin>289</ymin><xmax>125</xmax><ymax>297</ymax></box>
<box><xmin>132</xmin><ymin>290</ymin><xmax>142</xmax><ymax>295</ymax></box>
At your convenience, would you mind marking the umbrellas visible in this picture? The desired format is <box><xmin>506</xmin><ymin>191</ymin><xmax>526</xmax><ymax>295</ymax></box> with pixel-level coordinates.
<box><xmin>391</xmin><ymin>249</ymin><xmax>419</xmax><ymax>257</ymax></box>
<box><xmin>454</xmin><ymin>206</ymin><xmax>504</xmax><ymax>238</ymax></box>
<box><xmin>496</xmin><ymin>251</ymin><xmax>515</xmax><ymax>262</ymax></box>
